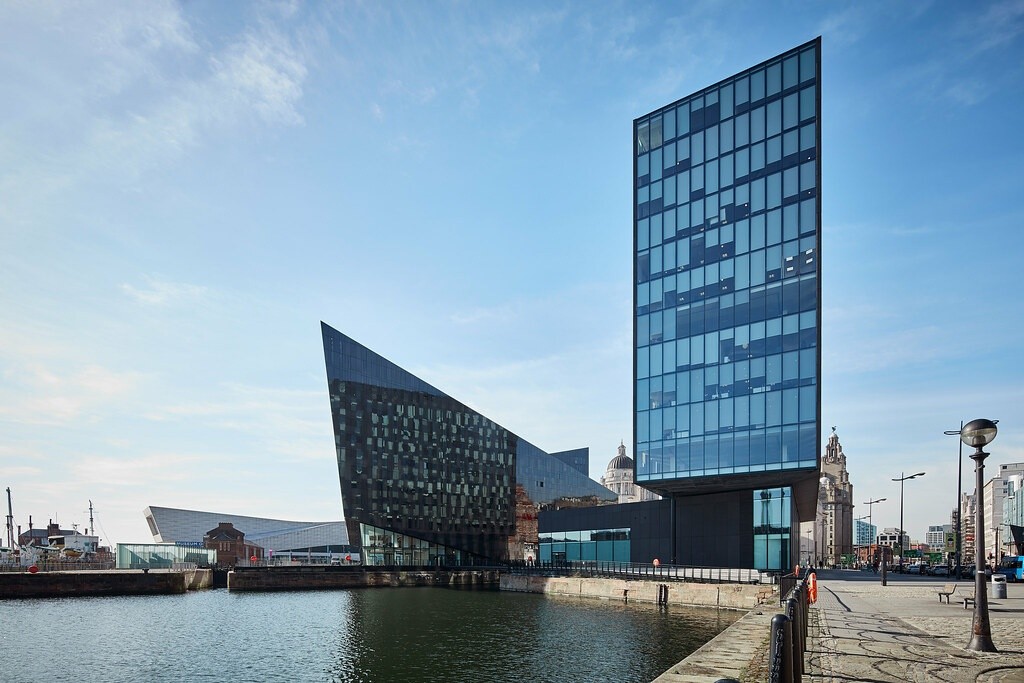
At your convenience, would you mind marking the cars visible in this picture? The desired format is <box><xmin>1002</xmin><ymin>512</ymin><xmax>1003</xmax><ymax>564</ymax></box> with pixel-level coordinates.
<box><xmin>832</xmin><ymin>554</ymin><xmax>1024</xmax><ymax>583</ymax></box>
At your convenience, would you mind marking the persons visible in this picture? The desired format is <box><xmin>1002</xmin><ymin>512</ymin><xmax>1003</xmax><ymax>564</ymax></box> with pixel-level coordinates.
<box><xmin>873</xmin><ymin>561</ymin><xmax>879</xmax><ymax>575</ymax></box>
<box><xmin>804</xmin><ymin>564</ymin><xmax>817</xmax><ymax>585</ymax></box>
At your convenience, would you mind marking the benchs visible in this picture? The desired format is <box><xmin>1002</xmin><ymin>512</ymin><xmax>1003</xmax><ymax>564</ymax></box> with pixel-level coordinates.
<box><xmin>937</xmin><ymin>584</ymin><xmax>957</xmax><ymax>604</ymax></box>
<box><xmin>958</xmin><ymin>586</ymin><xmax>988</xmax><ymax>609</ymax></box>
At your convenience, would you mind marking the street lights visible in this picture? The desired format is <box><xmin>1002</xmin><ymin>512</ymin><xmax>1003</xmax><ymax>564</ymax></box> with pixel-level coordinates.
<box><xmin>960</xmin><ymin>419</ymin><xmax>1000</xmax><ymax>652</ymax></box>
<box><xmin>892</xmin><ymin>471</ymin><xmax>926</xmax><ymax>574</ymax></box>
<box><xmin>854</xmin><ymin>516</ymin><xmax>872</xmax><ymax>558</ymax></box>
<box><xmin>999</xmin><ymin>523</ymin><xmax>1012</xmax><ymax>557</ymax></box>
<box><xmin>863</xmin><ymin>498</ymin><xmax>887</xmax><ymax>562</ymax></box>
<box><xmin>944</xmin><ymin>419</ymin><xmax>999</xmax><ymax>580</ymax></box>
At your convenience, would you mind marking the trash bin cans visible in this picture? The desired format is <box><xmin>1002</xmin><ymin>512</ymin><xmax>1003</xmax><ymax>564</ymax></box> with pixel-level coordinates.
<box><xmin>991</xmin><ymin>574</ymin><xmax>1007</xmax><ymax>599</ymax></box>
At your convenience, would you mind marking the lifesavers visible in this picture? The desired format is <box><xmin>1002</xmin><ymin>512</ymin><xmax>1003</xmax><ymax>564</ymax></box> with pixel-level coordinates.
<box><xmin>808</xmin><ymin>572</ymin><xmax>818</xmax><ymax>604</ymax></box>
<box><xmin>795</xmin><ymin>565</ymin><xmax>799</xmax><ymax>575</ymax></box>
